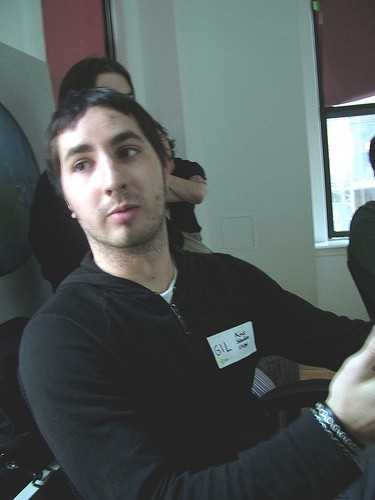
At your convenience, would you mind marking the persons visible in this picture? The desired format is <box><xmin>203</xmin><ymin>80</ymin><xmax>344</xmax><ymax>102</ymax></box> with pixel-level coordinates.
<box><xmin>19</xmin><ymin>87</ymin><xmax>375</xmax><ymax>500</ymax></box>
<box><xmin>29</xmin><ymin>58</ymin><xmax>184</xmax><ymax>294</ymax></box>
<box><xmin>346</xmin><ymin>135</ymin><xmax>375</xmax><ymax>321</ymax></box>
<box><xmin>153</xmin><ymin>122</ymin><xmax>206</xmax><ymax>243</ymax></box>
